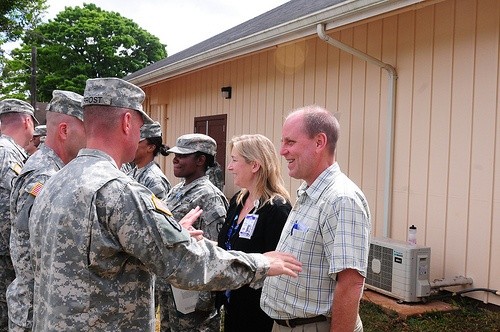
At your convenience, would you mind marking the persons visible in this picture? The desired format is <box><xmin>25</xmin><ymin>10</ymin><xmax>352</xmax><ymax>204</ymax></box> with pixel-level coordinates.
<box><xmin>218</xmin><ymin>134</ymin><xmax>293</xmax><ymax>332</ymax></box>
<box><xmin>259</xmin><ymin>104</ymin><xmax>372</xmax><ymax>332</ymax></box>
<box><xmin>160</xmin><ymin>133</ymin><xmax>230</xmax><ymax>332</ymax></box>
<box><xmin>32</xmin><ymin>125</ymin><xmax>48</xmax><ymax>148</ymax></box>
<box><xmin>126</xmin><ymin>121</ymin><xmax>172</xmax><ymax>318</ymax></box>
<box><xmin>0</xmin><ymin>99</ymin><xmax>40</xmax><ymax>332</ymax></box>
<box><xmin>28</xmin><ymin>77</ymin><xmax>303</xmax><ymax>332</ymax></box>
<box><xmin>205</xmin><ymin>161</ymin><xmax>224</xmax><ymax>193</ymax></box>
<box><xmin>6</xmin><ymin>90</ymin><xmax>86</xmax><ymax>332</ymax></box>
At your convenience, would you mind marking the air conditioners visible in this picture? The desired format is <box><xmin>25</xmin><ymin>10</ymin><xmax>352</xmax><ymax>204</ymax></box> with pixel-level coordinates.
<box><xmin>364</xmin><ymin>233</ymin><xmax>431</xmax><ymax>302</ymax></box>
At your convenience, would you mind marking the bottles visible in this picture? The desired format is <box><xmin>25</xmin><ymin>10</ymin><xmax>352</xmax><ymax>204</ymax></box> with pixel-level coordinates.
<box><xmin>408</xmin><ymin>224</ymin><xmax>417</xmax><ymax>248</ymax></box>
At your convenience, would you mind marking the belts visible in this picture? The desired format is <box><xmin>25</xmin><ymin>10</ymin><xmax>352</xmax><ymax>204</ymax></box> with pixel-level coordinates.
<box><xmin>274</xmin><ymin>315</ymin><xmax>327</xmax><ymax>328</ymax></box>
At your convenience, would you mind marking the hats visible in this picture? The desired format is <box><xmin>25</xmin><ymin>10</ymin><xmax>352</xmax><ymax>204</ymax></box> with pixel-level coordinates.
<box><xmin>33</xmin><ymin>125</ymin><xmax>47</xmax><ymax>136</ymax></box>
<box><xmin>166</xmin><ymin>134</ymin><xmax>217</xmax><ymax>158</ymax></box>
<box><xmin>0</xmin><ymin>99</ymin><xmax>39</xmax><ymax>124</ymax></box>
<box><xmin>45</xmin><ymin>90</ymin><xmax>84</xmax><ymax>122</ymax></box>
<box><xmin>139</xmin><ymin>121</ymin><xmax>163</xmax><ymax>142</ymax></box>
<box><xmin>82</xmin><ymin>78</ymin><xmax>154</xmax><ymax>124</ymax></box>
